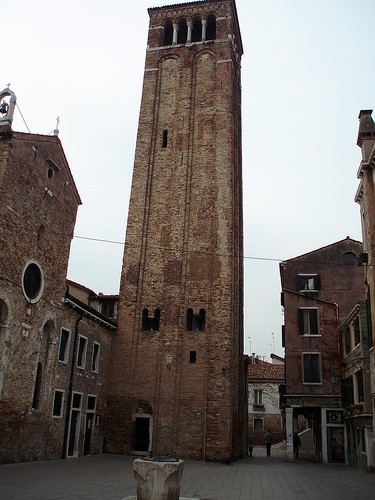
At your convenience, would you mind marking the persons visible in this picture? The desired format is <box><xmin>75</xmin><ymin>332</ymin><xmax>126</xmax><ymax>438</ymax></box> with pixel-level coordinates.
<box><xmin>294</xmin><ymin>432</ymin><xmax>302</xmax><ymax>458</ymax></box>
<box><xmin>263</xmin><ymin>429</ymin><xmax>273</xmax><ymax>456</ymax></box>
